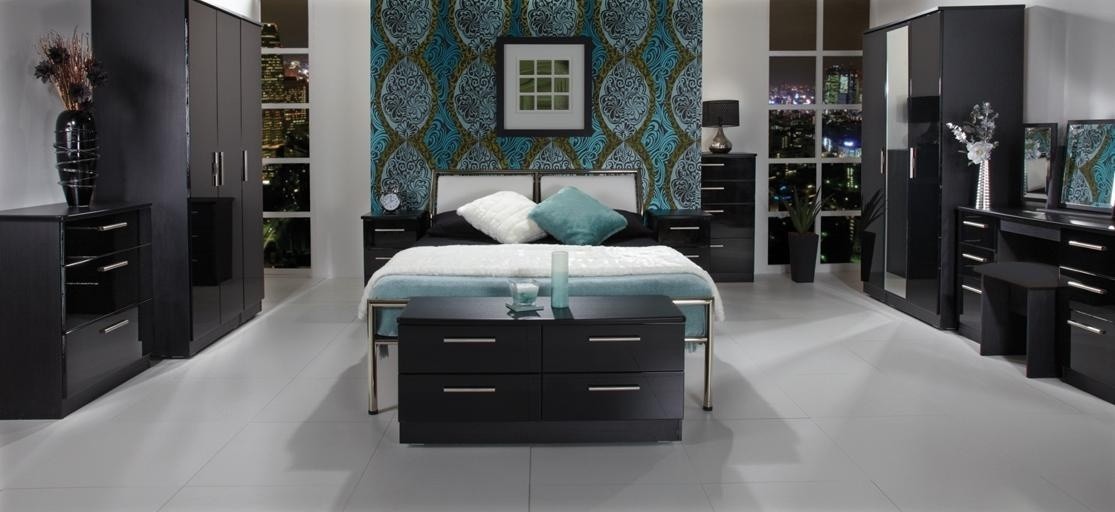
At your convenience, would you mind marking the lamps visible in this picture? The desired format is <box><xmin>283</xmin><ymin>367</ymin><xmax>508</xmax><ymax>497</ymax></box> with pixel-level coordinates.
<box><xmin>702</xmin><ymin>99</ymin><xmax>740</xmax><ymax>154</ymax></box>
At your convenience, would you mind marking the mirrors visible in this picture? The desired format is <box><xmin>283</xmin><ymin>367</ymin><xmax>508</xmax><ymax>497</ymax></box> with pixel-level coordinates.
<box><xmin>884</xmin><ymin>25</ymin><xmax>908</xmax><ymax>301</ymax></box>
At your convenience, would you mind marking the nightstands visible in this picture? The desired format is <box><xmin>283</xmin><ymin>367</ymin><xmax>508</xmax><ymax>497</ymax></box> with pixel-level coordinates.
<box><xmin>361</xmin><ymin>210</ymin><xmax>431</xmax><ymax>287</ymax></box>
<box><xmin>643</xmin><ymin>209</ymin><xmax>712</xmax><ymax>279</ymax></box>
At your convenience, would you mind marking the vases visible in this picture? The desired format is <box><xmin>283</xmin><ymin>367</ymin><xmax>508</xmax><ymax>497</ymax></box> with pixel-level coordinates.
<box><xmin>54</xmin><ymin>109</ymin><xmax>99</xmax><ymax>211</ymax></box>
<box><xmin>974</xmin><ymin>160</ymin><xmax>991</xmax><ymax>213</ymax></box>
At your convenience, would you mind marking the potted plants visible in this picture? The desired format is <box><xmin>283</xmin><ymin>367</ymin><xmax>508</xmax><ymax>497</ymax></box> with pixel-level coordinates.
<box><xmin>781</xmin><ymin>186</ymin><xmax>835</xmax><ymax>284</ymax></box>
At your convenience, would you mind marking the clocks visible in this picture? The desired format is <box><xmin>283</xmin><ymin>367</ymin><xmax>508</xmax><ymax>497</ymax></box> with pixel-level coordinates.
<box><xmin>380</xmin><ymin>178</ymin><xmax>401</xmax><ymax>215</ymax></box>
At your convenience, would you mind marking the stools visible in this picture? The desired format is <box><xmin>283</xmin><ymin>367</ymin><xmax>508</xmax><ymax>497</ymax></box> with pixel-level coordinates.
<box><xmin>971</xmin><ymin>261</ymin><xmax>1060</xmax><ymax>378</ymax></box>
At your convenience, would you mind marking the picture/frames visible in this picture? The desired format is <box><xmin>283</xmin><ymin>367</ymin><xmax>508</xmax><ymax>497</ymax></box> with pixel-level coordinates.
<box><xmin>1023</xmin><ymin>122</ymin><xmax>1057</xmax><ymax>209</ymax></box>
<box><xmin>495</xmin><ymin>36</ymin><xmax>593</xmax><ymax>138</ymax></box>
<box><xmin>1056</xmin><ymin>119</ymin><xmax>1115</xmax><ymax>214</ymax></box>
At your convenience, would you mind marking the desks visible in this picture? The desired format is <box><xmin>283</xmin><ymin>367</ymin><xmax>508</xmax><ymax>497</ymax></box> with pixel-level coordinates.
<box><xmin>953</xmin><ymin>205</ymin><xmax>1115</xmax><ymax>404</ymax></box>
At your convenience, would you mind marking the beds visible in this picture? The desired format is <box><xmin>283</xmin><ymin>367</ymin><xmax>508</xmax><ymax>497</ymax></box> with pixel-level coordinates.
<box><xmin>358</xmin><ymin>169</ymin><xmax>713</xmax><ymax>415</ymax></box>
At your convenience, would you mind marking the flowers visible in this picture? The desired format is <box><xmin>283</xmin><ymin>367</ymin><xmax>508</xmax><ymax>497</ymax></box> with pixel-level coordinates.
<box><xmin>946</xmin><ymin>102</ymin><xmax>1000</xmax><ymax>164</ymax></box>
<box><xmin>33</xmin><ymin>25</ymin><xmax>110</xmax><ymax>110</ymax></box>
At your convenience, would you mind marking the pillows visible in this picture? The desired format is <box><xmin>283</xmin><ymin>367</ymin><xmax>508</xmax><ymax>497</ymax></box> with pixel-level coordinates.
<box><xmin>529</xmin><ymin>186</ymin><xmax>628</xmax><ymax>245</ymax></box>
<box><xmin>455</xmin><ymin>191</ymin><xmax>548</xmax><ymax>243</ymax></box>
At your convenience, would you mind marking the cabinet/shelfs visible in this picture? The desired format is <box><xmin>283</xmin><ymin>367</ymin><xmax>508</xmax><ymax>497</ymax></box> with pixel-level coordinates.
<box><xmin>396</xmin><ymin>294</ymin><xmax>687</xmax><ymax>448</ymax></box>
<box><xmin>1</xmin><ymin>202</ymin><xmax>162</xmax><ymax>420</ymax></box>
<box><xmin>862</xmin><ymin>6</ymin><xmax>1024</xmax><ymax>329</ymax></box>
<box><xmin>91</xmin><ymin>1</ymin><xmax>264</xmax><ymax>361</ymax></box>
<box><xmin>701</xmin><ymin>153</ymin><xmax>756</xmax><ymax>283</ymax></box>
